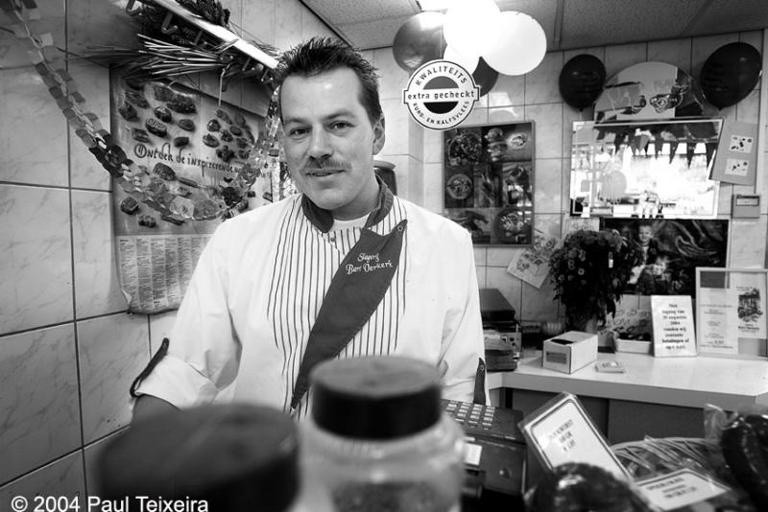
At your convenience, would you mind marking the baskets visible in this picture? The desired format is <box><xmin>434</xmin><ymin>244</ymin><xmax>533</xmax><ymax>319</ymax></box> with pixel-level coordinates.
<box><xmin>522</xmin><ymin>437</ymin><xmax>766</xmax><ymax>511</ymax></box>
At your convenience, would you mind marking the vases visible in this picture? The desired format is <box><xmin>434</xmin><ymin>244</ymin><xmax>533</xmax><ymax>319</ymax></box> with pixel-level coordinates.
<box><xmin>570</xmin><ymin>312</ymin><xmax>606</xmax><ymax>346</ymax></box>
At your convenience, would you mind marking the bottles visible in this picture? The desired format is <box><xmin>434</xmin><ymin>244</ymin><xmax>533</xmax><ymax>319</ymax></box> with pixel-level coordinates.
<box><xmin>626</xmin><ymin>139</ymin><xmax>708</xmax><ymax>185</ymax></box>
<box><xmin>301</xmin><ymin>356</ymin><xmax>465</xmax><ymax>512</ymax></box>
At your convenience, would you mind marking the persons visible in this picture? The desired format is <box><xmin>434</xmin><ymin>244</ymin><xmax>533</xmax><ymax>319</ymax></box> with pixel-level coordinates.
<box><xmin>631</xmin><ymin>222</ymin><xmax>666</xmax><ymax>296</ymax></box>
<box><xmin>128</xmin><ymin>35</ymin><xmax>493</xmax><ymax>425</ymax></box>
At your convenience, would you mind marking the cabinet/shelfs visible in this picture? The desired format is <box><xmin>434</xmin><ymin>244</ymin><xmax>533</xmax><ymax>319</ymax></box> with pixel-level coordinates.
<box><xmin>487</xmin><ymin>347</ymin><xmax>766</xmax><ymax>447</ymax></box>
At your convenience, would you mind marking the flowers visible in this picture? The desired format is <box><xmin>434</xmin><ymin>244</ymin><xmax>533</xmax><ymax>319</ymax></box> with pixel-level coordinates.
<box><xmin>548</xmin><ymin>230</ymin><xmax>646</xmax><ymax>326</ymax></box>
